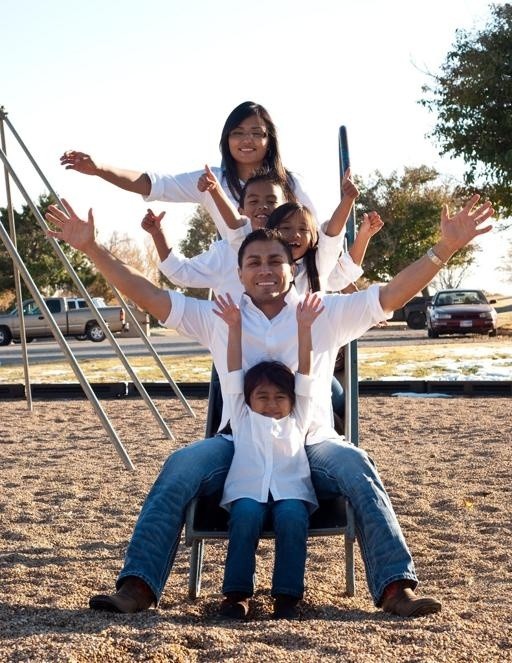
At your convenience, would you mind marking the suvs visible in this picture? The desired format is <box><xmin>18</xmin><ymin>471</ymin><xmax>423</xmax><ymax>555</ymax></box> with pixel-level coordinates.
<box><xmin>386</xmin><ymin>282</ymin><xmax>441</xmax><ymax>329</ymax></box>
<box><xmin>32</xmin><ymin>296</ymin><xmax>108</xmax><ymax>340</ymax></box>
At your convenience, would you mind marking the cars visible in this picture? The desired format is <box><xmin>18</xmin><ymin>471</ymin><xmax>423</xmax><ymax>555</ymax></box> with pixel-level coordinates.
<box><xmin>424</xmin><ymin>287</ymin><xmax>499</xmax><ymax>339</ymax></box>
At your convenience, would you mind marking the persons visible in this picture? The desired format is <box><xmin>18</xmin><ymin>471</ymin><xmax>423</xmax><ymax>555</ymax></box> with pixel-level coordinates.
<box><xmin>141</xmin><ymin>165</ymin><xmax>360</xmax><ymax>298</ymax></box>
<box><xmin>211</xmin><ymin>292</ymin><xmax>325</xmax><ymax>620</ymax></box>
<box><xmin>45</xmin><ymin>193</ymin><xmax>497</xmax><ymax>616</ymax></box>
<box><xmin>58</xmin><ymin>102</ymin><xmax>319</xmax><ymax>236</ymax></box>
<box><xmin>197</xmin><ymin>162</ymin><xmax>386</xmax><ymax>294</ymax></box>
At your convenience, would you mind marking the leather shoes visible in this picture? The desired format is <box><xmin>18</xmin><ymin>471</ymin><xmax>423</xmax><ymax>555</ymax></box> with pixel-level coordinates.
<box><xmin>384</xmin><ymin>582</ymin><xmax>442</xmax><ymax>616</ymax></box>
<box><xmin>88</xmin><ymin>575</ymin><xmax>159</xmax><ymax>613</ymax></box>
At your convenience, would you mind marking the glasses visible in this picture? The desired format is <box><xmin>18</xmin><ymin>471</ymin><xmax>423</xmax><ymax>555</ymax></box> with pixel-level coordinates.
<box><xmin>230</xmin><ymin>127</ymin><xmax>268</xmax><ymax>138</ymax></box>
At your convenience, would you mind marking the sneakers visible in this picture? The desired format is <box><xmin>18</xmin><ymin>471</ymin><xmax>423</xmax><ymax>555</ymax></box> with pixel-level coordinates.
<box><xmin>222</xmin><ymin>595</ymin><xmax>250</xmax><ymax>618</ymax></box>
<box><xmin>275</xmin><ymin>597</ymin><xmax>302</xmax><ymax>620</ymax></box>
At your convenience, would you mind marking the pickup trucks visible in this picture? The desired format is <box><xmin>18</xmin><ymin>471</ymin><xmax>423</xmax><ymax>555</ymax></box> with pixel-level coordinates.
<box><xmin>1</xmin><ymin>296</ymin><xmax>131</xmax><ymax>347</ymax></box>
